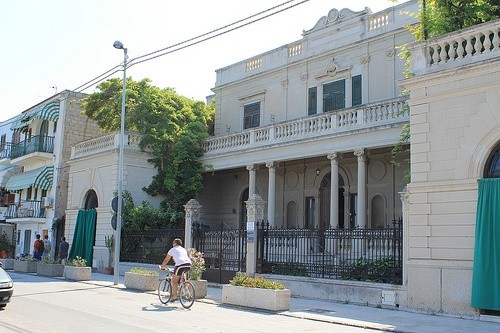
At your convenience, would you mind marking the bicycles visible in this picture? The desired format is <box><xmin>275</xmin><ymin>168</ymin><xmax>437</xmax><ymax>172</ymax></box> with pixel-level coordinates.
<box><xmin>156</xmin><ymin>266</ymin><xmax>196</xmax><ymax>309</ymax></box>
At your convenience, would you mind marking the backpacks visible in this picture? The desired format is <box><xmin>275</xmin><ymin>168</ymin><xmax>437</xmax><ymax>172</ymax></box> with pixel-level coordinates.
<box><xmin>37</xmin><ymin>240</ymin><xmax>44</xmax><ymax>253</ymax></box>
<box><xmin>43</xmin><ymin>240</ymin><xmax>51</xmax><ymax>252</ymax></box>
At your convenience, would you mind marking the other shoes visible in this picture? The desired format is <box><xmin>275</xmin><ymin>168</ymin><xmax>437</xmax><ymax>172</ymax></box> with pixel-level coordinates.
<box><xmin>170</xmin><ymin>297</ymin><xmax>178</xmax><ymax>302</ymax></box>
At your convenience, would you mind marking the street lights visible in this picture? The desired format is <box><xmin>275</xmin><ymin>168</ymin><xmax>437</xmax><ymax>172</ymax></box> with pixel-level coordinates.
<box><xmin>111</xmin><ymin>41</ymin><xmax>128</xmax><ymax>285</ymax></box>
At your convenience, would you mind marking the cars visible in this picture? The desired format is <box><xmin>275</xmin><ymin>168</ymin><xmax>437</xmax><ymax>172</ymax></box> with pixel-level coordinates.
<box><xmin>0</xmin><ymin>262</ymin><xmax>14</xmax><ymax>309</ymax></box>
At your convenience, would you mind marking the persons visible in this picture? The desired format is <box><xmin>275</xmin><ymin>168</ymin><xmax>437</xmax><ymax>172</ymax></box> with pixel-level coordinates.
<box><xmin>161</xmin><ymin>238</ymin><xmax>191</xmax><ymax>301</ymax></box>
<box><xmin>33</xmin><ymin>235</ymin><xmax>44</xmax><ymax>261</ymax></box>
<box><xmin>57</xmin><ymin>236</ymin><xmax>69</xmax><ymax>264</ymax></box>
<box><xmin>43</xmin><ymin>234</ymin><xmax>52</xmax><ymax>257</ymax></box>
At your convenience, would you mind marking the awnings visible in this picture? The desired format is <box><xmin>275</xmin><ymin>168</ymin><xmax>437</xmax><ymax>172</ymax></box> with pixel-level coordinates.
<box><xmin>0</xmin><ymin>165</ymin><xmax>24</xmax><ymax>188</ymax></box>
<box><xmin>6</xmin><ymin>165</ymin><xmax>54</xmax><ymax>194</ymax></box>
<box><xmin>11</xmin><ymin>99</ymin><xmax>60</xmax><ymax>133</ymax></box>
